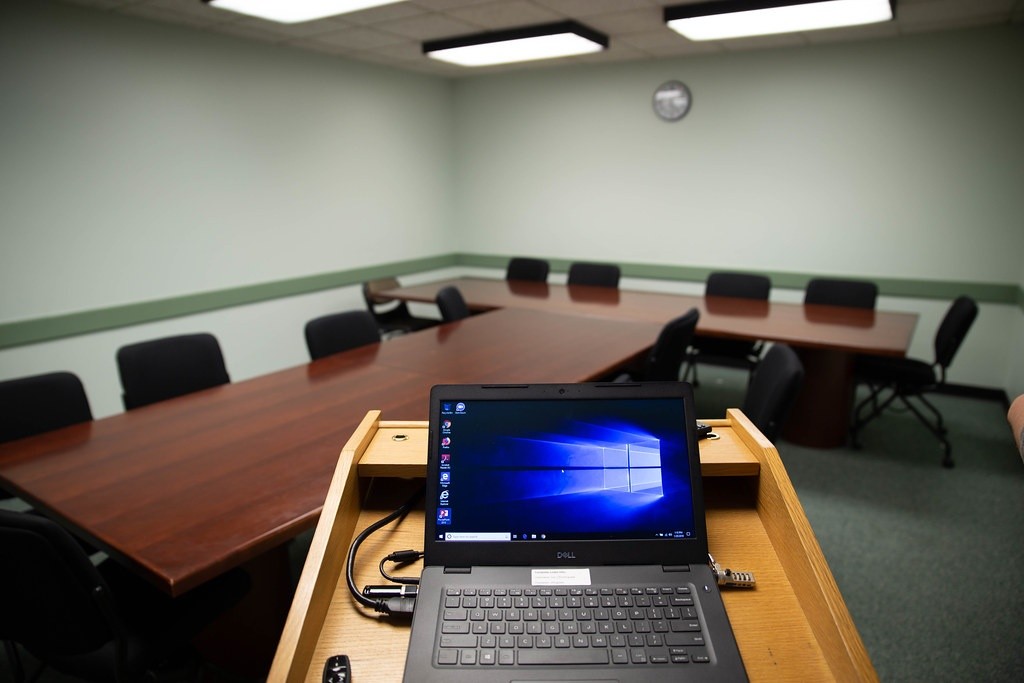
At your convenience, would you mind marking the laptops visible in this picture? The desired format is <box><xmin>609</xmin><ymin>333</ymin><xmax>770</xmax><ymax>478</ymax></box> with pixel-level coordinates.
<box><xmin>402</xmin><ymin>382</ymin><xmax>751</xmax><ymax>683</ymax></box>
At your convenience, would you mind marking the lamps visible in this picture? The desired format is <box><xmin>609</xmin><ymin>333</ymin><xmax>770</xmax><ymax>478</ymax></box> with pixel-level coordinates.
<box><xmin>422</xmin><ymin>19</ymin><xmax>610</xmax><ymax>68</ymax></box>
<box><xmin>662</xmin><ymin>0</ymin><xmax>896</xmax><ymax>42</ymax></box>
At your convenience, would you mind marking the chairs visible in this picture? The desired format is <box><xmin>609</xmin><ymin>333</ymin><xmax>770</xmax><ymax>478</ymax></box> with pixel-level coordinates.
<box><xmin>0</xmin><ymin>371</ymin><xmax>93</xmax><ymax>501</ymax></box>
<box><xmin>632</xmin><ymin>307</ymin><xmax>701</xmax><ymax>381</ymax></box>
<box><xmin>684</xmin><ymin>272</ymin><xmax>772</xmax><ymax>380</ymax></box>
<box><xmin>806</xmin><ymin>279</ymin><xmax>878</xmax><ymax>310</ymax></box>
<box><xmin>117</xmin><ymin>332</ymin><xmax>229</xmax><ymax>411</ymax></box>
<box><xmin>851</xmin><ymin>294</ymin><xmax>979</xmax><ymax>468</ymax></box>
<box><xmin>305</xmin><ymin>309</ymin><xmax>384</xmax><ymax>361</ymax></box>
<box><xmin>568</xmin><ymin>263</ymin><xmax>622</xmax><ymax>288</ymax></box>
<box><xmin>364</xmin><ymin>277</ymin><xmax>442</xmax><ymax>332</ymax></box>
<box><xmin>507</xmin><ymin>258</ymin><xmax>550</xmax><ymax>282</ymax></box>
<box><xmin>0</xmin><ymin>504</ymin><xmax>252</xmax><ymax>683</ymax></box>
<box><xmin>437</xmin><ymin>286</ymin><xmax>471</xmax><ymax>321</ymax></box>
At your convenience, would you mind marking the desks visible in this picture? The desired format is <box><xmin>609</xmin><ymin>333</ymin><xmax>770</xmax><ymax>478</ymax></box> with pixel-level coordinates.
<box><xmin>0</xmin><ymin>306</ymin><xmax>664</xmax><ymax>683</ymax></box>
<box><xmin>381</xmin><ymin>275</ymin><xmax>920</xmax><ymax>450</ymax></box>
